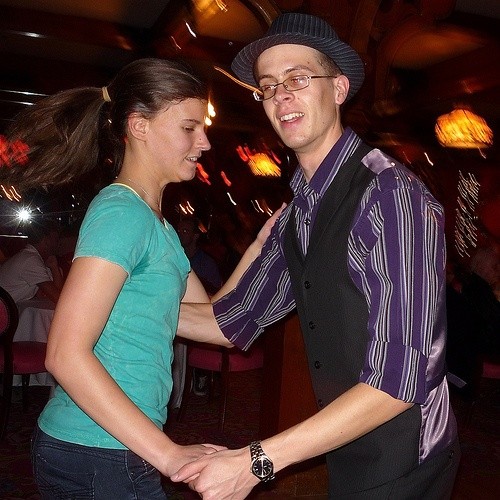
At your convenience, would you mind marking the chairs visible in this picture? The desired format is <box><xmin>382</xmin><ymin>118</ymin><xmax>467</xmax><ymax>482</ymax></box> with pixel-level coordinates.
<box><xmin>177</xmin><ymin>339</ymin><xmax>266</xmax><ymax>434</ymax></box>
<box><xmin>0</xmin><ymin>285</ymin><xmax>60</xmax><ymax>443</ymax></box>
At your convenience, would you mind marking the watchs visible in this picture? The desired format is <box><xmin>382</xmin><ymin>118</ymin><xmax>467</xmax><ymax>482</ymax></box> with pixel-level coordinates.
<box><xmin>249</xmin><ymin>440</ymin><xmax>276</xmax><ymax>483</ymax></box>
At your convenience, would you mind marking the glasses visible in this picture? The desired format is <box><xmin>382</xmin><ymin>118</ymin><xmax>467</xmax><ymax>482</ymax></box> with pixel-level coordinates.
<box><xmin>253</xmin><ymin>75</ymin><xmax>337</xmax><ymax>101</ymax></box>
<box><xmin>177</xmin><ymin>229</ymin><xmax>190</xmax><ymax>234</ymax></box>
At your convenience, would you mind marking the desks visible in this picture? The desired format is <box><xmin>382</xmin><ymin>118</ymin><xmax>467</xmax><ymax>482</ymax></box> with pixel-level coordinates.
<box><xmin>14</xmin><ymin>298</ymin><xmax>55</xmax><ymax>386</ymax></box>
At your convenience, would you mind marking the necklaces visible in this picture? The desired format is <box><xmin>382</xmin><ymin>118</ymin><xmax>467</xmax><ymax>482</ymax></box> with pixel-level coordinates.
<box><xmin>116</xmin><ymin>175</ymin><xmax>165</xmax><ymax>224</ymax></box>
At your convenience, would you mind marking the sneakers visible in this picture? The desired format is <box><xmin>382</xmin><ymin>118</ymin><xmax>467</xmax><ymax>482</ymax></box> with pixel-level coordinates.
<box><xmin>193</xmin><ymin>368</ymin><xmax>209</xmax><ymax>396</ymax></box>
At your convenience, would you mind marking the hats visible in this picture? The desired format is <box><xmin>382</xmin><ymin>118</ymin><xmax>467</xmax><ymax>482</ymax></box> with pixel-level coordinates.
<box><xmin>231</xmin><ymin>13</ymin><xmax>366</xmax><ymax>103</ymax></box>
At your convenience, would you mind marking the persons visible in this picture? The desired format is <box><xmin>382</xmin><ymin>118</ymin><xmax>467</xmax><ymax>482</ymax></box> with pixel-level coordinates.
<box><xmin>446</xmin><ymin>253</ymin><xmax>499</xmax><ymax>407</ymax></box>
<box><xmin>7</xmin><ymin>55</ymin><xmax>286</xmax><ymax>500</ymax></box>
<box><xmin>169</xmin><ymin>12</ymin><xmax>462</xmax><ymax>500</ymax></box>
<box><xmin>0</xmin><ymin>225</ymin><xmax>80</xmax><ymax>305</ymax></box>
<box><xmin>192</xmin><ymin>230</ymin><xmax>243</xmax><ymax>295</ymax></box>
